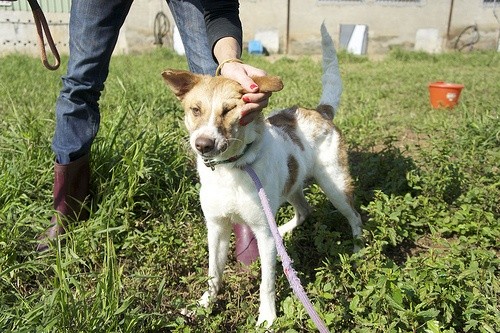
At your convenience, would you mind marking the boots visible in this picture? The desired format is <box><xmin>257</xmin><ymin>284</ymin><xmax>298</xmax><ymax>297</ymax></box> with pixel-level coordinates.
<box><xmin>34</xmin><ymin>152</ymin><xmax>97</xmax><ymax>252</ymax></box>
<box><xmin>232</xmin><ymin>223</ymin><xmax>260</xmax><ymax>266</ymax></box>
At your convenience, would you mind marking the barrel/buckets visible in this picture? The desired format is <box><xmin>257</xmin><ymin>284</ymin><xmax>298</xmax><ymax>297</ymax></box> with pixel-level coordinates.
<box><xmin>428</xmin><ymin>82</ymin><xmax>464</xmax><ymax>110</ymax></box>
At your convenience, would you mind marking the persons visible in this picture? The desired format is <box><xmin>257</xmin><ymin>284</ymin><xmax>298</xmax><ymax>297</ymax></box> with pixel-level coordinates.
<box><xmin>35</xmin><ymin>0</ymin><xmax>272</xmax><ymax>276</ymax></box>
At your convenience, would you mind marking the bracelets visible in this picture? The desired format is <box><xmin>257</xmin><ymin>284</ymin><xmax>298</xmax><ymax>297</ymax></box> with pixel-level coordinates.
<box><xmin>216</xmin><ymin>58</ymin><xmax>243</xmax><ymax>76</ymax></box>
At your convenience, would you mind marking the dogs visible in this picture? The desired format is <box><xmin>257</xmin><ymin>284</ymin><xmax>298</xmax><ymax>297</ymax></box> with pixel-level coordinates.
<box><xmin>158</xmin><ymin>15</ymin><xmax>366</xmax><ymax>333</ymax></box>
